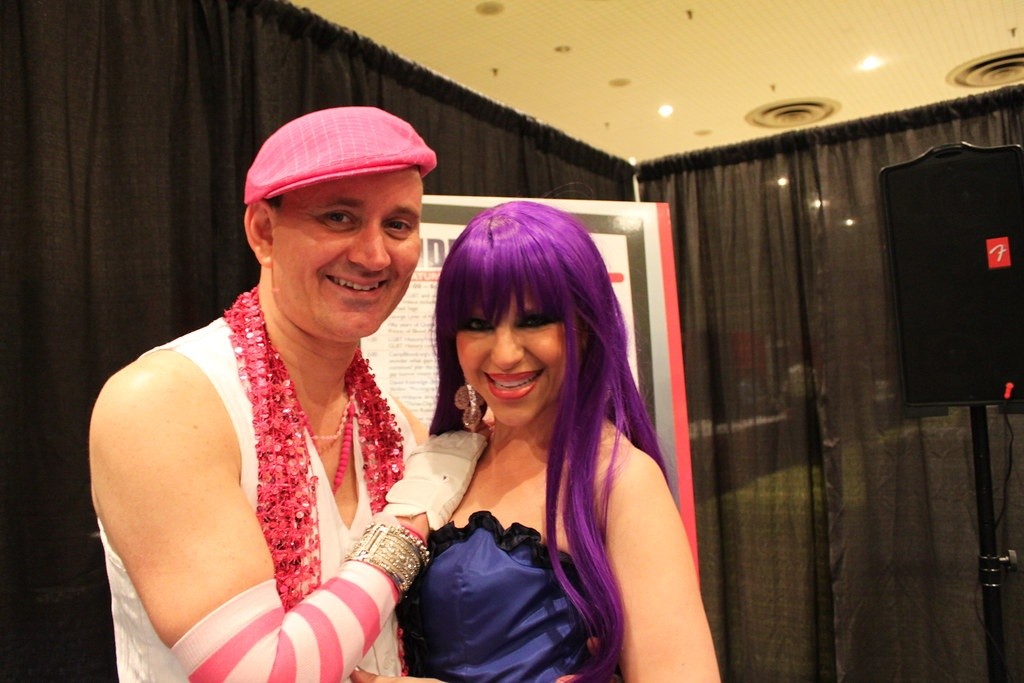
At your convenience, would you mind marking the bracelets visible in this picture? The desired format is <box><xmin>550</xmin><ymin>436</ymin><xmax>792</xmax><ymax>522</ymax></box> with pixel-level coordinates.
<box><xmin>345</xmin><ymin>523</ymin><xmax>431</xmax><ymax>593</ymax></box>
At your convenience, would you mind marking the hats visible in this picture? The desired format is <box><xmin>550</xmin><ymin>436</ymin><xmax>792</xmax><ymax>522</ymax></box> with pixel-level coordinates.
<box><xmin>243</xmin><ymin>105</ymin><xmax>440</xmax><ymax>202</ymax></box>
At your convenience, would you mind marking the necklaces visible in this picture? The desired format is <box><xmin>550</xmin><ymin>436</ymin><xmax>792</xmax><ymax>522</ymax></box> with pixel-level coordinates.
<box><xmin>279</xmin><ymin>364</ymin><xmax>355</xmax><ymax>495</ymax></box>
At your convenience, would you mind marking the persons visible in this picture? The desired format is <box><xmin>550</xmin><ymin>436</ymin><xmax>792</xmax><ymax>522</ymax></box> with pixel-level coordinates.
<box><xmin>349</xmin><ymin>202</ymin><xmax>721</xmax><ymax>683</ymax></box>
<box><xmin>90</xmin><ymin>106</ymin><xmax>495</xmax><ymax>683</ymax></box>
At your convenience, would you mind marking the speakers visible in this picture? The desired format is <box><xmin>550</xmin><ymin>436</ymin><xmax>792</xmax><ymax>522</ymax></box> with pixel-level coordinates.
<box><xmin>879</xmin><ymin>142</ymin><xmax>1024</xmax><ymax>406</ymax></box>
